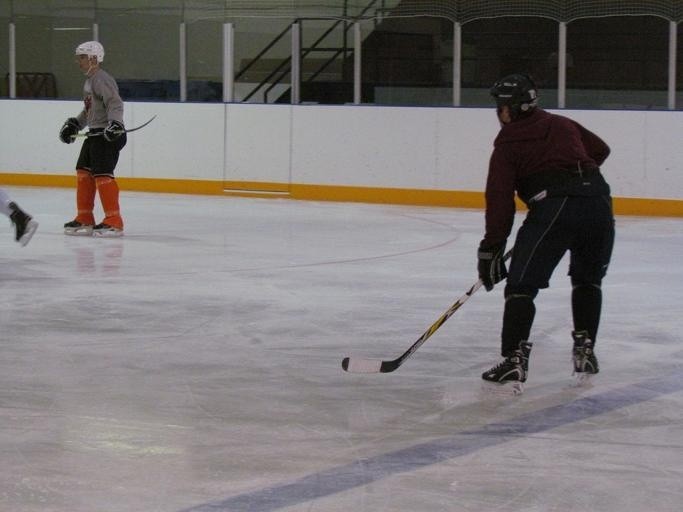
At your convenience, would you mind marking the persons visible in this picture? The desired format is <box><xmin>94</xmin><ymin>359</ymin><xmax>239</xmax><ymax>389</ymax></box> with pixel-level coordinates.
<box><xmin>0</xmin><ymin>188</ymin><xmax>42</xmax><ymax>248</ymax></box>
<box><xmin>475</xmin><ymin>72</ymin><xmax>612</xmax><ymax>400</ymax></box>
<box><xmin>58</xmin><ymin>40</ymin><xmax>128</xmax><ymax>238</ymax></box>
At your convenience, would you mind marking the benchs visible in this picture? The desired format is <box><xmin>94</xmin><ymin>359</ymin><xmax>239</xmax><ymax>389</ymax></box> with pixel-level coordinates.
<box><xmin>353</xmin><ymin>0</ymin><xmax>683</xmax><ymax>91</ymax></box>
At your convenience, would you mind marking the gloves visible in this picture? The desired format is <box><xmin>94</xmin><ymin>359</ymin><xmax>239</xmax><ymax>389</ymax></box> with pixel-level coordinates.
<box><xmin>478</xmin><ymin>236</ymin><xmax>509</xmax><ymax>293</ymax></box>
<box><xmin>59</xmin><ymin>117</ymin><xmax>82</xmax><ymax>144</ymax></box>
<box><xmin>103</xmin><ymin>120</ymin><xmax>125</xmax><ymax>142</ymax></box>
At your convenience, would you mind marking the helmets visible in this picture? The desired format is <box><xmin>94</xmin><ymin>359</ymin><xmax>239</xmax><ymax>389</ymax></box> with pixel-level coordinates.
<box><xmin>75</xmin><ymin>41</ymin><xmax>106</xmax><ymax>65</ymax></box>
<box><xmin>489</xmin><ymin>73</ymin><xmax>539</xmax><ymax>113</ymax></box>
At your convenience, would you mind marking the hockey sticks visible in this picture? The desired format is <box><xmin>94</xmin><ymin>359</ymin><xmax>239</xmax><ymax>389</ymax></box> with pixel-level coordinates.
<box><xmin>341</xmin><ymin>248</ymin><xmax>514</xmax><ymax>374</ymax></box>
<box><xmin>70</xmin><ymin>115</ymin><xmax>156</xmax><ymax>137</ymax></box>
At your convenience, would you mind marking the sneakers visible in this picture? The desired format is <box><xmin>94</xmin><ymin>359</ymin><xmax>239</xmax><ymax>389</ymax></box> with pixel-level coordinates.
<box><xmin>481</xmin><ymin>341</ymin><xmax>534</xmax><ymax>384</ymax></box>
<box><xmin>571</xmin><ymin>331</ymin><xmax>600</xmax><ymax>377</ymax></box>
<box><xmin>93</xmin><ymin>221</ymin><xmax>122</xmax><ymax>232</ymax></box>
<box><xmin>8</xmin><ymin>202</ymin><xmax>33</xmax><ymax>241</ymax></box>
<box><xmin>63</xmin><ymin>217</ymin><xmax>90</xmax><ymax>229</ymax></box>
